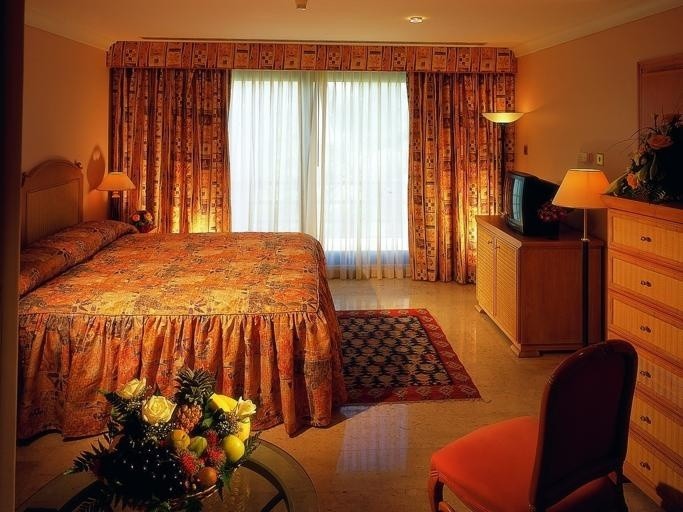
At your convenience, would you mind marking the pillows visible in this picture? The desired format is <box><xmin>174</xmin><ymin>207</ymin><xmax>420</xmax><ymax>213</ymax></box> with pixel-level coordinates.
<box><xmin>18</xmin><ymin>245</ymin><xmax>68</xmax><ymax>301</ymax></box>
<box><xmin>31</xmin><ymin>217</ymin><xmax>140</xmax><ymax>274</ymax></box>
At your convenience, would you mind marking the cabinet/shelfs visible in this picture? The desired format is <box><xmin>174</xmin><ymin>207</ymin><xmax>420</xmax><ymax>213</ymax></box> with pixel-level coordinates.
<box><xmin>474</xmin><ymin>212</ymin><xmax>606</xmax><ymax>359</ymax></box>
<box><xmin>598</xmin><ymin>192</ymin><xmax>683</xmax><ymax>511</ymax></box>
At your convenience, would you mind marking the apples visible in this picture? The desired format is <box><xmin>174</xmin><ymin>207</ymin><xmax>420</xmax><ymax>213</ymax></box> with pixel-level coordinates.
<box><xmin>223</xmin><ymin>434</ymin><xmax>247</xmax><ymax>463</ymax></box>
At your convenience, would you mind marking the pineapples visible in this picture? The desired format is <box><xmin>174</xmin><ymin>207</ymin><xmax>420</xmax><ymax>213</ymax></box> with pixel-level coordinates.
<box><xmin>171</xmin><ymin>370</ymin><xmax>217</xmax><ymax>434</ymax></box>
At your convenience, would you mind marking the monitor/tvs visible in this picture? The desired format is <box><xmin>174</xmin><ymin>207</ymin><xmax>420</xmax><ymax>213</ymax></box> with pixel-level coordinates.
<box><xmin>503</xmin><ymin>171</ymin><xmax>576</xmax><ymax>238</ymax></box>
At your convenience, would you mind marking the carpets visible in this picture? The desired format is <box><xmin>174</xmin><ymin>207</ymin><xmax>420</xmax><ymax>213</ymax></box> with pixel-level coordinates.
<box><xmin>333</xmin><ymin>305</ymin><xmax>484</xmax><ymax>410</ymax></box>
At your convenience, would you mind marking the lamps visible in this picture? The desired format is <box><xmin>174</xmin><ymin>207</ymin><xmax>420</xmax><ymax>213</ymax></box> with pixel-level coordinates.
<box><xmin>480</xmin><ymin>110</ymin><xmax>523</xmax><ymax>125</ymax></box>
<box><xmin>96</xmin><ymin>171</ymin><xmax>137</xmax><ymax>221</ymax></box>
<box><xmin>550</xmin><ymin>168</ymin><xmax>614</xmax><ymax>348</ymax></box>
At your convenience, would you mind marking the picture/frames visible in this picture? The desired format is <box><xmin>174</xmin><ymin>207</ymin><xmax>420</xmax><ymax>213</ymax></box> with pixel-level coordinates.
<box><xmin>636</xmin><ymin>53</ymin><xmax>683</xmax><ymax>157</ymax></box>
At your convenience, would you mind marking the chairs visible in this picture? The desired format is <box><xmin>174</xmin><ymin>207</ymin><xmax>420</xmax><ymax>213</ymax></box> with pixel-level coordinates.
<box><xmin>427</xmin><ymin>338</ymin><xmax>638</xmax><ymax>511</ymax></box>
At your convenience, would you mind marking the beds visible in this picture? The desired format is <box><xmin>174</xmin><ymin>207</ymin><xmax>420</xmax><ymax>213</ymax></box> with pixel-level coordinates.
<box><xmin>19</xmin><ymin>157</ymin><xmax>351</xmax><ymax>439</ymax></box>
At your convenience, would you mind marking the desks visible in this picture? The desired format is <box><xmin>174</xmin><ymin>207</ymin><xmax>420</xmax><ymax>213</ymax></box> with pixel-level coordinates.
<box><xmin>13</xmin><ymin>431</ymin><xmax>320</xmax><ymax>512</ymax></box>
